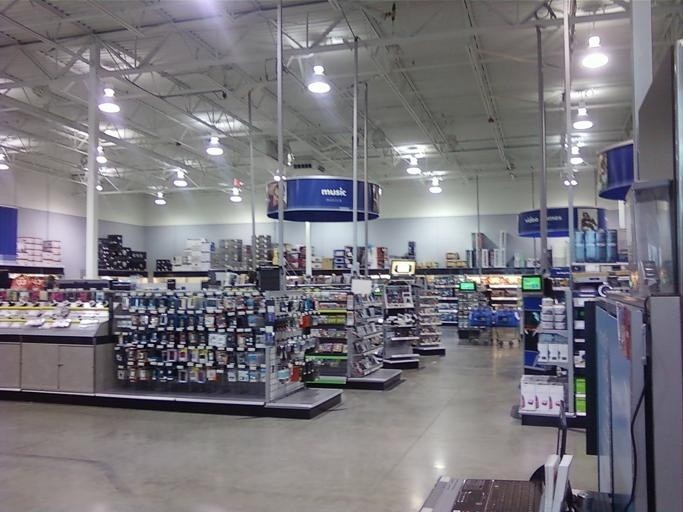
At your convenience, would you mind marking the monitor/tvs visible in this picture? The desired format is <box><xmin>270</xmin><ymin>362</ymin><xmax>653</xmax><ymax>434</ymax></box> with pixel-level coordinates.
<box><xmin>520</xmin><ymin>274</ymin><xmax>545</xmax><ymax>297</ymax></box>
<box><xmin>459</xmin><ymin>281</ymin><xmax>476</xmax><ymax>292</ymax></box>
<box><xmin>389</xmin><ymin>259</ymin><xmax>417</xmax><ymax>280</ymax></box>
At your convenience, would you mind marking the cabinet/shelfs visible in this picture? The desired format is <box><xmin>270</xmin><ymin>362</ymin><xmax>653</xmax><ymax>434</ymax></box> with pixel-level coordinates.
<box><xmin>0</xmin><ymin>341</ymin><xmax>115</xmax><ymax>393</ymax></box>
<box><xmin>518</xmin><ymin>286</ymin><xmax>577</xmax><ymax>426</ymax></box>
<box><xmin>303</xmin><ymin>271</ymin><xmax>524</xmax><ymax>390</ymax></box>
